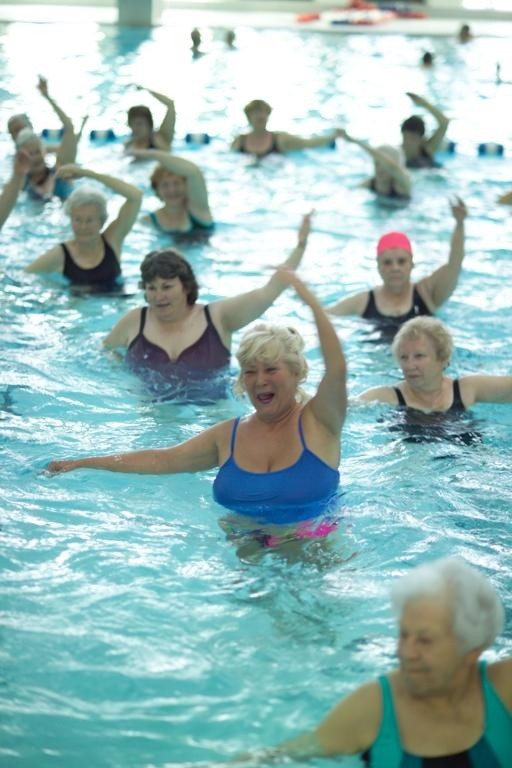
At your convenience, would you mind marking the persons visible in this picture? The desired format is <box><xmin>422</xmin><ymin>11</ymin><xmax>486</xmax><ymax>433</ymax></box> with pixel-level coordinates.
<box><xmin>43</xmin><ymin>260</ymin><xmax>350</xmax><ymax>566</ymax></box>
<box><xmin>221</xmin><ymin>549</ymin><xmax>512</xmax><ymax>768</ymax></box>
<box><xmin>322</xmin><ymin>191</ymin><xmax>468</xmax><ymax>337</ymax></box>
<box><xmin>341</xmin><ymin>313</ymin><xmax>511</xmax><ymax>450</ymax></box>
<box><xmin>23</xmin><ymin>160</ymin><xmax>143</xmax><ymax>299</ymax></box>
<box><xmin>97</xmin><ymin>203</ymin><xmax>316</xmax><ymax>409</ymax></box>
<box><xmin>1</xmin><ymin>20</ymin><xmax>512</xmax><ymax>250</ymax></box>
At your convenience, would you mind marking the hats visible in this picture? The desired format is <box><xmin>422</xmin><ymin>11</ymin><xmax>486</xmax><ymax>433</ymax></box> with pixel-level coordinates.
<box><xmin>377</xmin><ymin>231</ymin><xmax>412</xmax><ymax>255</ymax></box>
